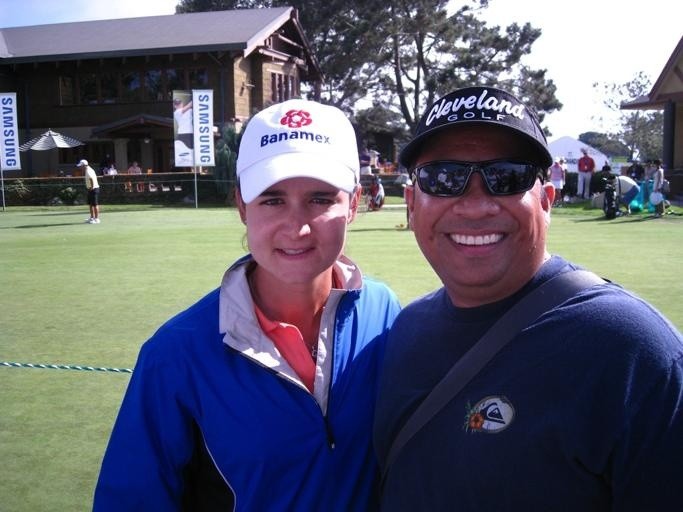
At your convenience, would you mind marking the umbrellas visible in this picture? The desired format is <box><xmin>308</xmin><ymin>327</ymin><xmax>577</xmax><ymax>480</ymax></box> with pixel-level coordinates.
<box><xmin>18</xmin><ymin>128</ymin><xmax>87</xmax><ymax>175</ymax></box>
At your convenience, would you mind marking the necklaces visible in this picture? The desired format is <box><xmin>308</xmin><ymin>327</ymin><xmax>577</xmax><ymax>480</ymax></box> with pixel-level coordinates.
<box><xmin>245</xmin><ymin>275</ymin><xmax>319</xmax><ymax>360</ymax></box>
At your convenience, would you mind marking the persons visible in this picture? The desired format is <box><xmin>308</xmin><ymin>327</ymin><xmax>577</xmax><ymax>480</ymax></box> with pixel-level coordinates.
<box><xmin>90</xmin><ymin>94</ymin><xmax>404</xmax><ymax>512</ymax></box>
<box><xmin>174</xmin><ymin>96</ymin><xmax>193</xmax><ymax>149</ymax></box>
<box><xmin>548</xmin><ymin>138</ymin><xmax>669</xmax><ymax>221</ymax></box>
<box><xmin>365</xmin><ymin>84</ymin><xmax>681</xmax><ymax>509</ymax></box>
<box><xmin>127</xmin><ymin>161</ymin><xmax>142</xmax><ymax>174</ymax></box>
<box><xmin>74</xmin><ymin>158</ymin><xmax>102</xmax><ymax>225</ymax></box>
<box><xmin>363</xmin><ymin>175</ymin><xmax>386</xmax><ymax>211</ymax></box>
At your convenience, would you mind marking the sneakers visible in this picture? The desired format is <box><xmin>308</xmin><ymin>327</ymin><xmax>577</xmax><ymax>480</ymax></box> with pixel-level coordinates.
<box><xmin>84</xmin><ymin>217</ymin><xmax>101</xmax><ymax>224</ymax></box>
<box><xmin>653</xmin><ymin>211</ymin><xmax>666</xmax><ymax>218</ymax></box>
<box><xmin>621</xmin><ymin>210</ymin><xmax>632</xmax><ymax>216</ymax></box>
<box><xmin>552</xmin><ymin>202</ymin><xmax>562</xmax><ymax>208</ymax></box>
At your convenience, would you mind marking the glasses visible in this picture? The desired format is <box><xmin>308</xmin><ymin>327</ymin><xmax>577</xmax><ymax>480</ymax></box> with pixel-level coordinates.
<box><xmin>412</xmin><ymin>154</ymin><xmax>546</xmax><ymax>197</ymax></box>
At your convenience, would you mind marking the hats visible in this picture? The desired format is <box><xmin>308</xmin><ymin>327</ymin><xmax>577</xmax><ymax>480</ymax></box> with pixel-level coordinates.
<box><xmin>400</xmin><ymin>85</ymin><xmax>553</xmax><ymax>173</ymax></box>
<box><xmin>233</xmin><ymin>96</ymin><xmax>363</xmax><ymax>203</ymax></box>
<box><xmin>76</xmin><ymin>159</ymin><xmax>88</xmax><ymax>167</ymax></box>
<box><xmin>554</xmin><ymin>157</ymin><xmax>564</xmax><ymax>163</ymax></box>
